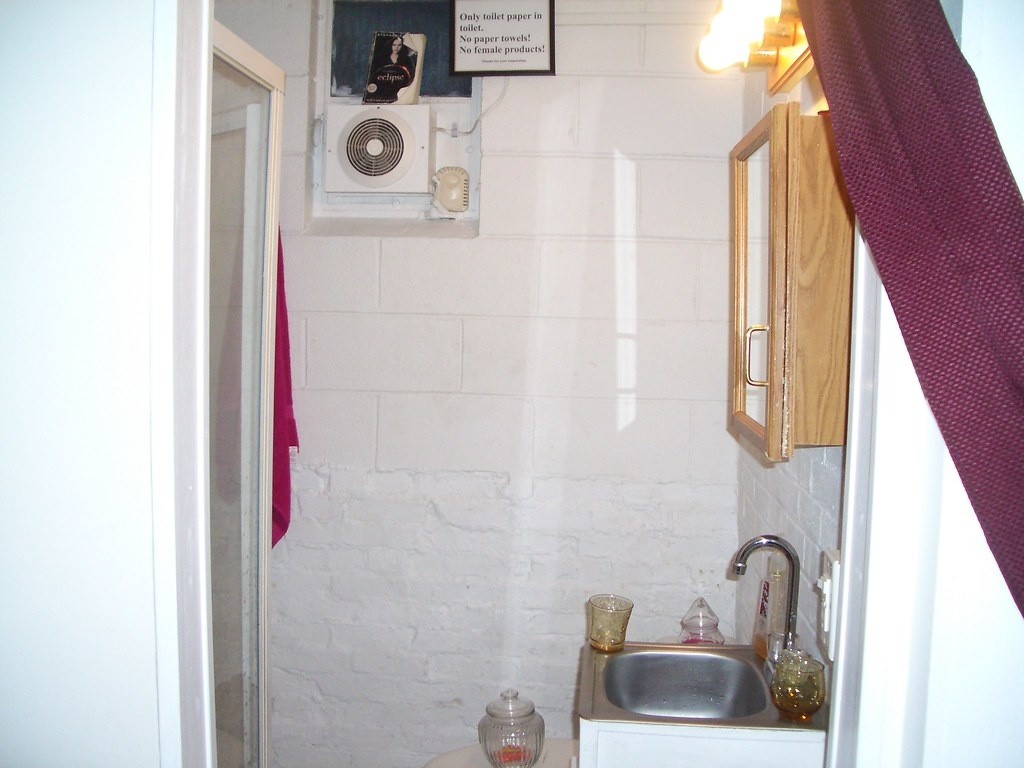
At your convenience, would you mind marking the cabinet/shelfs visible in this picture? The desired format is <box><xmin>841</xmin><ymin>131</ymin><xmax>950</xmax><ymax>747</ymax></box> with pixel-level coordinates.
<box><xmin>728</xmin><ymin>102</ymin><xmax>854</xmax><ymax>463</ymax></box>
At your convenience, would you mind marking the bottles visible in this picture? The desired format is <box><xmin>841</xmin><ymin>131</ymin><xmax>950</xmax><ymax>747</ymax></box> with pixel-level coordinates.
<box><xmin>477</xmin><ymin>688</ymin><xmax>546</xmax><ymax>768</ymax></box>
<box><xmin>753</xmin><ymin>552</ymin><xmax>789</xmax><ymax>659</ymax></box>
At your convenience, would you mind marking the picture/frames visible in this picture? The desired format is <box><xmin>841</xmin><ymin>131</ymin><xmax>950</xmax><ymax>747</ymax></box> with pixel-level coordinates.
<box><xmin>448</xmin><ymin>0</ymin><xmax>556</xmax><ymax>77</ymax></box>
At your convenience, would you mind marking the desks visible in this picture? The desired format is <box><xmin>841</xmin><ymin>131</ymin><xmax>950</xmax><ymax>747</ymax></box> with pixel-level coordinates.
<box><xmin>424</xmin><ymin>739</ymin><xmax>578</xmax><ymax>767</ymax></box>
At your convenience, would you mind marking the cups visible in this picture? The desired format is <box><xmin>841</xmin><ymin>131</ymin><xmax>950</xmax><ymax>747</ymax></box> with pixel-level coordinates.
<box><xmin>588</xmin><ymin>593</ymin><xmax>634</xmax><ymax>651</ymax></box>
<box><xmin>771</xmin><ymin>657</ymin><xmax>826</xmax><ymax>724</ymax></box>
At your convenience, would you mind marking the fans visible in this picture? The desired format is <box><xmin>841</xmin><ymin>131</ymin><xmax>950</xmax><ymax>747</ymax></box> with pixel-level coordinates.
<box><xmin>327</xmin><ymin>104</ymin><xmax>436</xmax><ymax>196</ymax></box>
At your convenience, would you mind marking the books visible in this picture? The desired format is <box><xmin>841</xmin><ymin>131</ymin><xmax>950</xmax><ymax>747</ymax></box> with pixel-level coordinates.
<box><xmin>363</xmin><ymin>31</ymin><xmax>426</xmax><ymax>105</ymax></box>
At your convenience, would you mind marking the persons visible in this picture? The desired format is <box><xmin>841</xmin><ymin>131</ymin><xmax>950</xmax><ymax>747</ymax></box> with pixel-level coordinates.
<box><xmin>373</xmin><ymin>36</ymin><xmax>413</xmax><ymax>100</ymax></box>
